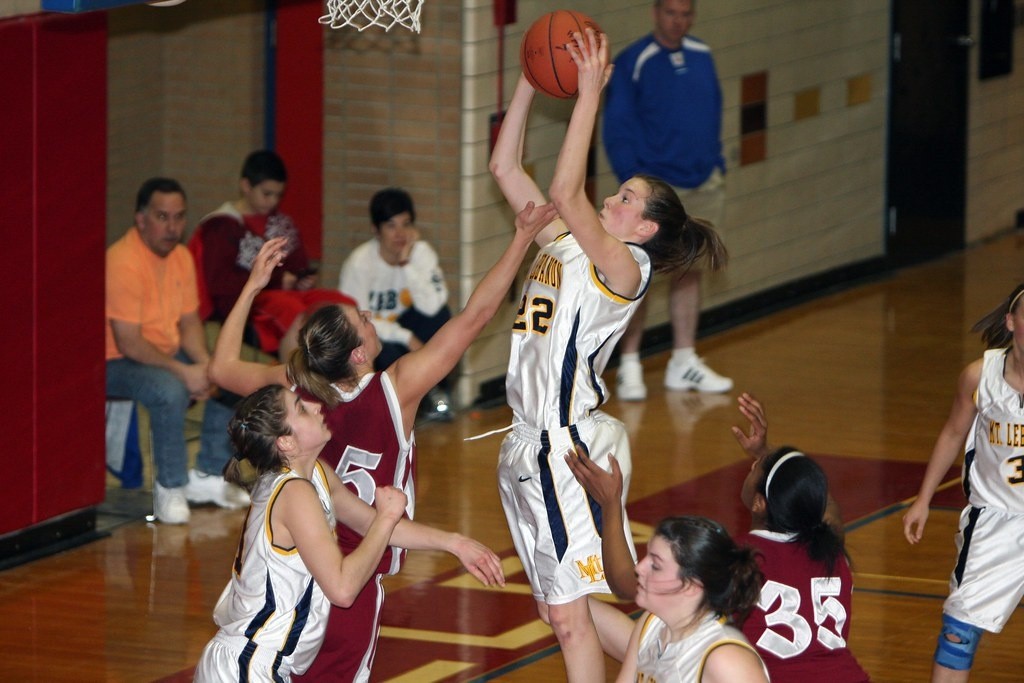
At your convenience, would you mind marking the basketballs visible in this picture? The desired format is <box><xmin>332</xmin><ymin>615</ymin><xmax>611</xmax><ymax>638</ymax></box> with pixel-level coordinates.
<box><xmin>520</xmin><ymin>9</ymin><xmax>608</xmax><ymax>98</ymax></box>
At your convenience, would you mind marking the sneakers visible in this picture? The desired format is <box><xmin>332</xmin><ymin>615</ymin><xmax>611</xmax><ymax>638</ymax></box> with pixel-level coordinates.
<box><xmin>616</xmin><ymin>361</ymin><xmax>647</xmax><ymax>398</ymax></box>
<box><xmin>184</xmin><ymin>469</ymin><xmax>251</xmax><ymax>507</ymax></box>
<box><xmin>664</xmin><ymin>359</ymin><xmax>732</xmax><ymax>392</ymax></box>
<box><xmin>153</xmin><ymin>479</ymin><xmax>189</xmax><ymax>523</ymax></box>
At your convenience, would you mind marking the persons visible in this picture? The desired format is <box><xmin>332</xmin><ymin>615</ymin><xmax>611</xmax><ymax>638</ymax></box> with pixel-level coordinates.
<box><xmin>102</xmin><ymin>176</ymin><xmax>253</xmax><ymax>525</ymax></box>
<box><xmin>564</xmin><ymin>392</ymin><xmax>877</xmax><ymax>682</ymax></box>
<box><xmin>598</xmin><ymin>0</ymin><xmax>735</xmax><ymax>401</ymax></box>
<box><xmin>335</xmin><ymin>188</ymin><xmax>458</xmax><ymax>421</ymax></box>
<box><xmin>182</xmin><ymin>149</ymin><xmax>319</xmax><ymax>483</ymax></box>
<box><xmin>489</xmin><ymin>27</ymin><xmax>729</xmax><ymax>681</ymax></box>
<box><xmin>210</xmin><ymin>197</ymin><xmax>560</xmax><ymax>683</ymax></box>
<box><xmin>898</xmin><ymin>280</ymin><xmax>1024</xmax><ymax>683</ymax></box>
<box><xmin>613</xmin><ymin>513</ymin><xmax>774</xmax><ymax>682</ymax></box>
<box><xmin>189</xmin><ymin>384</ymin><xmax>507</xmax><ymax>683</ymax></box>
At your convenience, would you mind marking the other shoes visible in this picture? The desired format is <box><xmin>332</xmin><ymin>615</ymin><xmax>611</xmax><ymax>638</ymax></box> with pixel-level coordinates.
<box><xmin>421</xmin><ymin>387</ymin><xmax>456</xmax><ymax>420</ymax></box>
